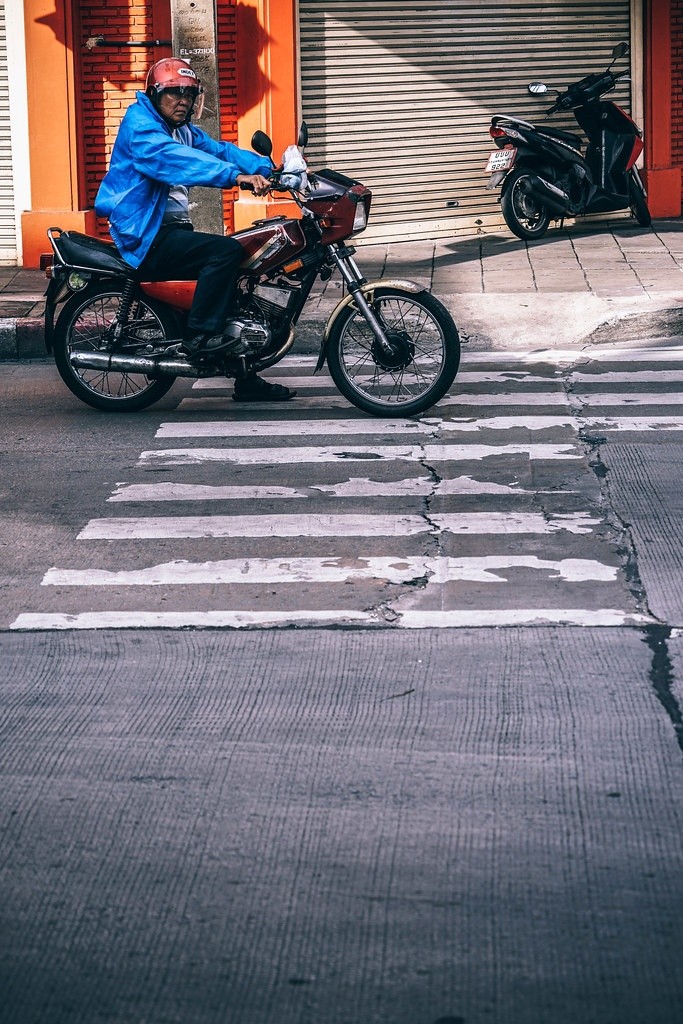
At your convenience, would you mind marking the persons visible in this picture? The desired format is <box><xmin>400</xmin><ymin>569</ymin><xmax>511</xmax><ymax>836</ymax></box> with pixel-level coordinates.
<box><xmin>94</xmin><ymin>58</ymin><xmax>299</xmax><ymax>400</ymax></box>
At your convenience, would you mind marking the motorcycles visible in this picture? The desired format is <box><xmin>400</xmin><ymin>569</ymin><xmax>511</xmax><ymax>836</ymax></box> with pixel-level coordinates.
<box><xmin>482</xmin><ymin>42</ymin><xmax>653</xmax><ymax>242</ymax></box>
<box><xmin>39</xmin><ymin>120</ymin><xmax>463</xmax><ymax>418</ymax></box>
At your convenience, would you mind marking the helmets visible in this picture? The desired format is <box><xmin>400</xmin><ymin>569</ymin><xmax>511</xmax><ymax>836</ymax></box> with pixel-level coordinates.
<box><xmin>146</xmin><ymin>56</ymin><xmax>203</xmax><ymax>129</ymax></box>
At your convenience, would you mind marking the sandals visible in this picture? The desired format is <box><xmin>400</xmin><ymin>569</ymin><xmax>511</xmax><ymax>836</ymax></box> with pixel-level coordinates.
<box><xmin>182</xmin><ymin>332</ymin><xmax>240</xmax><ymax>362</ymax></box>
<box><xmin>232</xmin><ymin>376</ymin><xmax>296</xmax><ymax>402</ymax></box>
